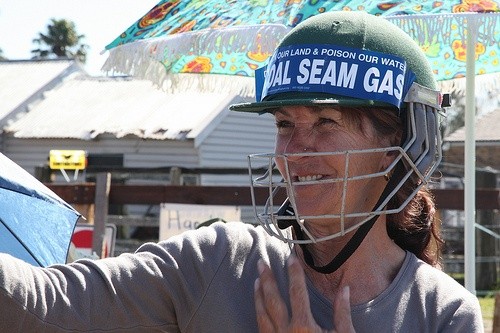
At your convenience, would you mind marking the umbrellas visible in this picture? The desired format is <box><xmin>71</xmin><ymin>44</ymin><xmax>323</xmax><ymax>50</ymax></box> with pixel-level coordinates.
<box><xmin>101</xmin><ymin>0</ymin><xmax>500</xmax><ymax>295</ymax></box>
<box><xmin>0</xmin><ymin>150</ymin><xmax>87</xmax><ymax>268</ymax></box>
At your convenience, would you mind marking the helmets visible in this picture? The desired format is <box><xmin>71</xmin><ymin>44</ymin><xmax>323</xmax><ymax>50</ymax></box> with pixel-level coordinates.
<box><xmin>229</xmin><ymin>10</ymin><xmax>442</xmax><ymax>184</ymax></box>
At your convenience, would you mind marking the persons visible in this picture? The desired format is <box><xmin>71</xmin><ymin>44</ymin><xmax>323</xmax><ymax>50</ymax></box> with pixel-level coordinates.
<box><xmin>0</xmin><ymin>10</ymin><xmax>485</xmax><ymax>333</ymax></box>
